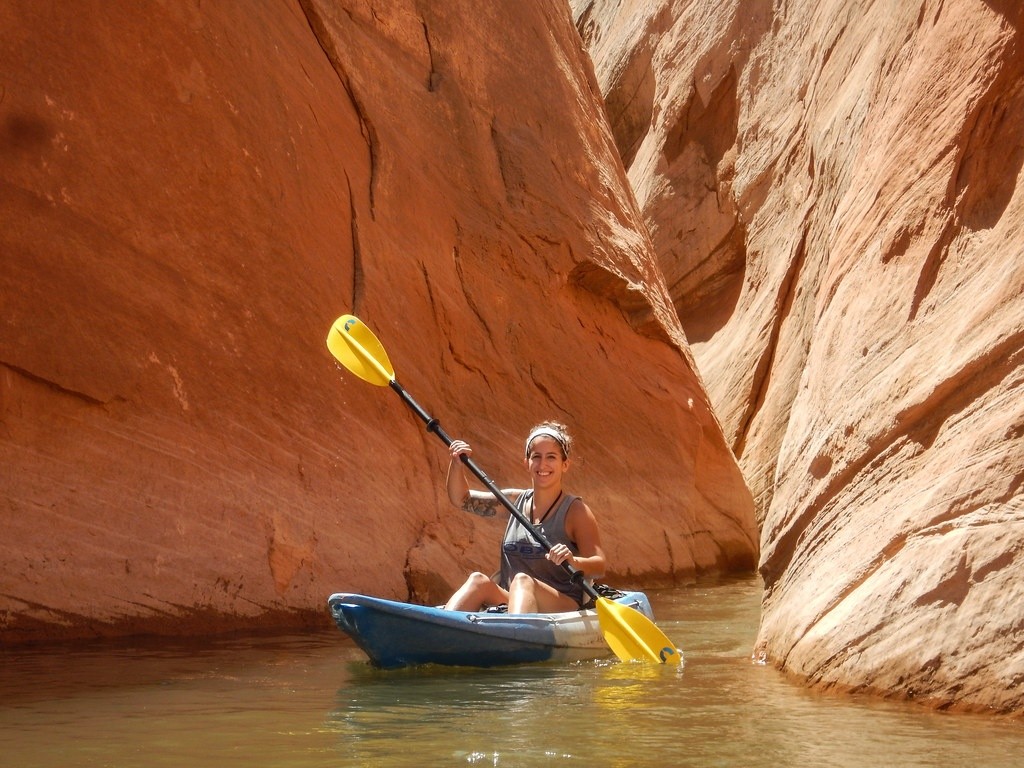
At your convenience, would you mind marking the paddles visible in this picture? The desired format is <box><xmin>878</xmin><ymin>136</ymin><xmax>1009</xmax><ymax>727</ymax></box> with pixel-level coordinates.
<box><xmin>324</xmin><ymin>312</ymin><xmax>682</xmax><ymax>669</ymax></box>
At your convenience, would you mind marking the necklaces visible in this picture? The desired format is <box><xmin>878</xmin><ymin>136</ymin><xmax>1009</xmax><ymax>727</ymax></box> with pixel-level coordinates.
<box><xmin>530</xmin><ymin>489</ymin><xmax>563</xmax><ymax>525</ymax></box>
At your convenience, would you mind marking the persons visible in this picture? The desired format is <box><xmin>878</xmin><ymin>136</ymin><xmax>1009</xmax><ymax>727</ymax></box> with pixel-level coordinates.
<box><xmin>443</xmin><ymin>419</ymin><xmax>607</xmax><ymax>614</ymax></box>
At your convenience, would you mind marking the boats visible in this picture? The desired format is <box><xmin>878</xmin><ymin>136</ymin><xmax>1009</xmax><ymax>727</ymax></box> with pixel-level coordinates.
<box><xmin>328</xmin><ymin>587</ymin><xmax>655</xmax><ymax>669</ymax></box>
